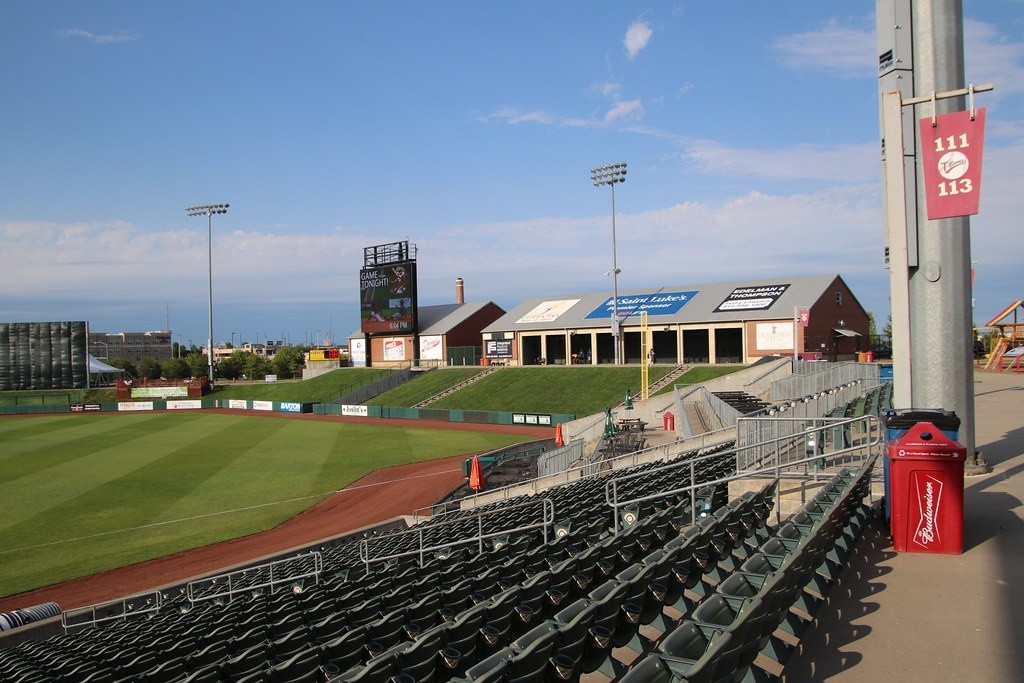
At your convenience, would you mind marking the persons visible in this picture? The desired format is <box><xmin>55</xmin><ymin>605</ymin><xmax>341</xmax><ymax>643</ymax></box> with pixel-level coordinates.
<box><xmin>648</xmin><ymin>349</ymin><xmax>655</xmax><ymax>365</ymax></box>
<box><xmin>832</xmin><ymin>343</ymin><xmax>839</xmax><ymax>362</ymax></box>
<box><xmin>82</xmin><ymin>401</ymin><xmax>85</xmax><ymax>412</ymax></box>
<box><xmin>387</xmin><ymin>300</ymin><xmax>407</xmax><ymax>321</ymax></box>
<box><xmin>365</xmin><ymin>298</ymin><xmax>384</xmax><ymax>322</ymax></box>
<box><xmin>572</xmin><ymin>348</ymin><xmax>591</xmax><ymax>363</ymax></box>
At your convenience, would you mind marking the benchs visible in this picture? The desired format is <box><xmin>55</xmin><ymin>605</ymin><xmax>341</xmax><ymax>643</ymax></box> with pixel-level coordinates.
<box><xmin>735</xmin><ymin>407</ymin><xmax>763</xmax><ymax>409</ymax></box>
<box><xmin>715</xmin><ymin>393</ymin><xmax>749</xmax><ymax>395</ymax></box>
<box><xmin>616</xmin><ymin>421</ymin><xmax>648</xmax><ymax>425</ymax></box>
<box><xmin>724</xmin><ymin>399</ymin><xmax>763</xmax><ymax>401</ymax></box>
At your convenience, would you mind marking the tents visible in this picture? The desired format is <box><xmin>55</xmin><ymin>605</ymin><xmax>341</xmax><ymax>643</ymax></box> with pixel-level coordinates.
<box><xmin>89</xmin><ymin>353</ymin><xmax>125</xmax><ymax>387</ymax></box>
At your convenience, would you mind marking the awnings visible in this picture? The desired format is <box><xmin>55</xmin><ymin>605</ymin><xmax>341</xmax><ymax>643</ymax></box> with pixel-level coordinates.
<box><xmin>833</xmin><ymin>328</ymin><xmax>862</xmax><ymax>337</ymax></box>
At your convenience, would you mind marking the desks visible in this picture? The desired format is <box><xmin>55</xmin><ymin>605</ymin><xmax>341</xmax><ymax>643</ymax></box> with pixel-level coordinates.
<box><xmin>619</xmin><ymin>418</ymin><xmax>642</xmax><ymax>431</ymax></box>
<box><xmin>711</xmin><ymin>391</ymin><xmax>772</xmax><ymax>414</ymax></box>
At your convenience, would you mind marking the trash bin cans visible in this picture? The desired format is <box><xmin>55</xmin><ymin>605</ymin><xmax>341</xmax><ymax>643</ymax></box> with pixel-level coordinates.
<box><xmin>480</xmin><ymin>357</ymin><xmax>491</xmax><ymax>366</ymax></box>
<box><xmin>854</xmin><ymin>350</ymin><xmax>867</xmax><ymax>363</ymax></box>
<box><xmin>880</xmin><ymin>408</ymin><xmax>961</xmax><ymax>532</ymax></box>
<box><xmin>865</xmin><ymin>350</ymin><xmax>875</xmax><ymax>364</ymax></box>
<box><xmin>885</xmin><ymin>421</ymin><xmax>968</xmax><ymax>555</ymax></box>
<box><xmin>663</xmin><ymin>412</ymin><xmax>675</xmax><ymax>431</ymax></box>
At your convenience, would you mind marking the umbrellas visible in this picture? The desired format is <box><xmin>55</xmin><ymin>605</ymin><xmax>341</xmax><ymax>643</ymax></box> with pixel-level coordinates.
<box><xmin>604</xmin><ymin>406</ymin><xmax>617</xmax><ymax>440</ymax></box>
<box><xmin>624</xmin><ymin>388</ymin><xmax>633</xmax><ymax>418</ymax></box>
<box><xmin>469</xmin><ymin>454</ymin><xmax>484</xmax><ymax>495</ymax></box>
<box><xmin>554</xmin><ymin>423</ymin><xmax>563</xmax><ymax>447</ymax></box>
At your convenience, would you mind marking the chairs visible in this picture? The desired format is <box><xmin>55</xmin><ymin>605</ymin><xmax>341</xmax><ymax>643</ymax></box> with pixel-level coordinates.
<box><xmin>0</xmin><ymin>443</ymin><xmax>879</xmax><ymax>683</ymax></box>
